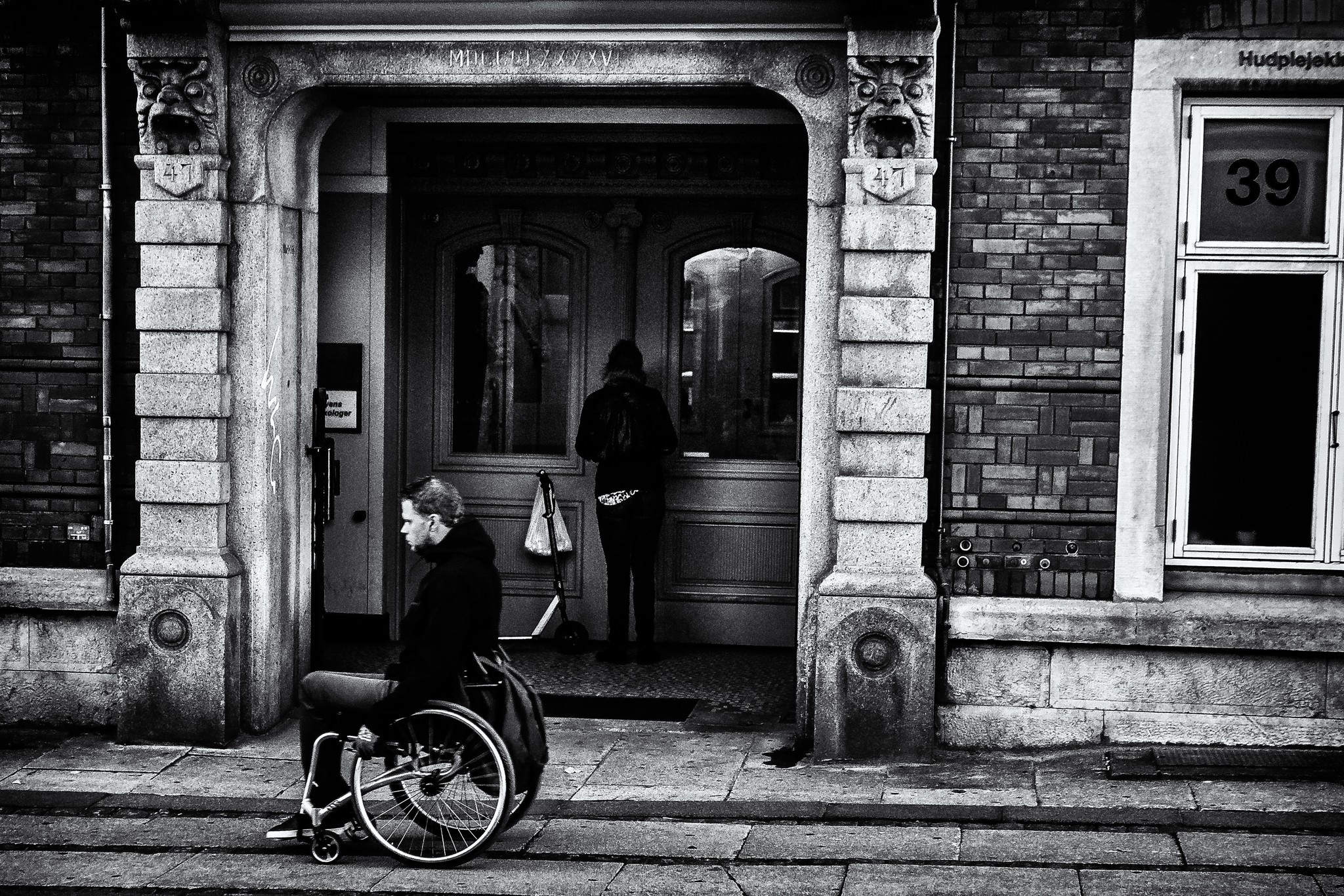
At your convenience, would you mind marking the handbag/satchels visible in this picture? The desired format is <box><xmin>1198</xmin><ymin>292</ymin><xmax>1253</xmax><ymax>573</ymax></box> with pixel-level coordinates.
<box><xmin>524</xmin><ymin>472</ymin><xmax>572</xmax><ymax>556</ymax></box>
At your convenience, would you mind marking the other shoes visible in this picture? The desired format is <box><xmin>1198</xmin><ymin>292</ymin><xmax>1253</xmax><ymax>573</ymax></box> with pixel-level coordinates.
<box><xmin>632</xmin><ymin>645</ymin><xmax>661</xmax><ymax>664</ymax></box>
<box><xmin>597</xmin><ymin>643</ymin><xmax>629</xmax><ymax>664</ymax></box>
<box><xmin>266</xmin><ymin>791</ymin><xmax>354</xmax><ymax>838</ymax></box>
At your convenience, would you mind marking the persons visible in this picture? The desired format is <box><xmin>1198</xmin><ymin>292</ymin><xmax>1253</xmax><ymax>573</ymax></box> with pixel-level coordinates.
<box><xmin>265</xmin><ymin>474</ymin><xmax>505</xmax><ymax>841</ymax></box>
<box><xmin>575</xmin><ymin>340</ymin><xmax>681</xmax><ymax>666</ymax></box>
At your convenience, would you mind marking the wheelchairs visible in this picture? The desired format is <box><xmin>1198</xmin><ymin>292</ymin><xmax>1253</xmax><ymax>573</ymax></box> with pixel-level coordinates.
<box><xmin>272</xmin><ymin>644</ymin><xmax>545</xmax><ymax>871</ymax></box>
